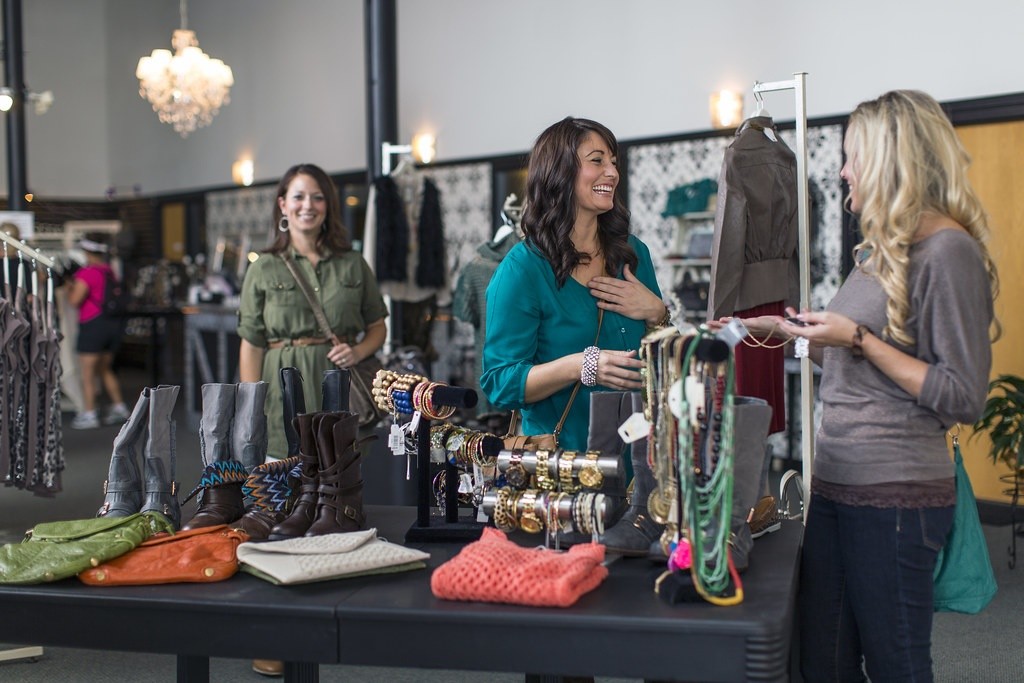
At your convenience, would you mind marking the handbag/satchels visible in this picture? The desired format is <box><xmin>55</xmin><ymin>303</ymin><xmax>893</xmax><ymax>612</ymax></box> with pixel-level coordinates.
<box><xmin>236</xmin><ymin>529</ymin><xmax>431</xmax><ymax>585</ymax></box>
<box><xmin>431</xmin><ymin>527</ymin><xmax>608</xmax><ymax>608</ymax></box>
<box><xmin>0</xmin><ymin>512</ymin><xmax>175</xmax><ymax>583</ymax></box>
<box><xmin>80</xmin><ymin>524</ymin><xmax>251</xmax><ymax>585</ymax></box>
<box><xmin>483</xmin><ymin>435</ymin><xmax>557</xmax><ymax>479</ymax></box>
<box><xmin>934</xmin><ymin>443</ymin><xmax>998</xmax><ymax>614</ymax></box>
<box><xmin>348</xmin><ymin>357</ymin><xmax>389</xmax><ymax>430</ymax></box>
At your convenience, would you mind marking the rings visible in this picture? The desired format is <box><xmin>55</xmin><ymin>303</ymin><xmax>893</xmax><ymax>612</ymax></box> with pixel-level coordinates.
<box><xmin>342</xmin><ymin>358</ymin><xmax>346</xmax><ymax>363</ymax></box>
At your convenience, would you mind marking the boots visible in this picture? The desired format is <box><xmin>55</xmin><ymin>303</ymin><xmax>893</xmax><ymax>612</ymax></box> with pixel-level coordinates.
<box><xmin>95</xmin><ymin>387</ymin><xmax>150</xmax><ymax>517</ymax></box>
<box><xmin>321</xmin><ymin>368</ymin><xmax>352</xmax><ymax>413</ymax></box>
<box><xmin>234</xmin><ymin>456</ymin><xmax>301</xmax><ymax>539</ymax></box>
<box><xmin>550</xmin><ymin>391</ymin><xmax>631</xmax><ymax>549</ymax></box>
<box><xmin>650</xmin><ymin>397</ymin><xmax>772</xmax><ymax>570</ymax></box>
<box><xmin>268</xmin><ymin>412</ymin><xmax>315</xmax><ymax>538</ymax></box>
<box><xmin>180</xmin><ymin>460</ymin><xmax>249</xmax><ymax>531</ymax></box>
<box><xmin>280</xmin><ymin>365</ymin><xmax>306</xmax><ymax>457</ymax></box>
<box><xmin>601</xmin><ymin>393</ymin><xmax>667</xmax><ymax>553</ymax></box>
<box><xmin>196</xmin><ymin>383</ymin><xmax>235</xmax><ymax>504</ymax></box>
<box><xmin>140</xmin><ymin>385</ymin><xmax>180</xmax><ymax>524</ymax></box>
<box><xmin>306</xmin><ymin>414</ymin><xmax>380</xmax><ymax>534</ymax></box>
<box><xmin>229</xmin><ymin>382</ymin><xmax>269</xmax><ymax>473</ymax></box>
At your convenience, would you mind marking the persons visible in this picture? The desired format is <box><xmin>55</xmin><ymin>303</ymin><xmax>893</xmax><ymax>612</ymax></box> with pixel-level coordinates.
<box><xmin>0</xmin><ymin>222</ymin><xmax>33</xmax><ymax>303</ymax></box>
<box><xmin>480</xmin><ymin>118</ymin><xmax>671</xmax><ymax>488</ymax></box>
<box><xmin>707</xmin><ymin>89</ymin><xmax>1002</xmax><ymax>683</ymax></box>
<box><xmin>236</xmin><ymin>164</ymin><xmax>389</xmax><ymax>675</ymax></box>
<box><xmin>64</xmin><ymin>232</ymin><xmax>131</xmax><ymax>429</ymax></box>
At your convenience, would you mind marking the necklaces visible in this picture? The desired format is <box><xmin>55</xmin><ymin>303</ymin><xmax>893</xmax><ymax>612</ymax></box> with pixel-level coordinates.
<box><xmin>635</xmin><ymin>329</ymin><xmax>743</xmax><ymax>605</ymax></box>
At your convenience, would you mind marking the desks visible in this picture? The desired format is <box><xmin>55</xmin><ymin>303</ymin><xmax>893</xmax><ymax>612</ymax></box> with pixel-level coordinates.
<box><xmin>0</xmin><ymin>507</ymin><xmax>806</xmax><ymax>683</ymax></box>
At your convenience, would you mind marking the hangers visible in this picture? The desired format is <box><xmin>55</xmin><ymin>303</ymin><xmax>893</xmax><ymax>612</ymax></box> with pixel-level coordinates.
<box><xmin>743</xmin><ymin>81</ymin><xmax>774</xmax><ymax>122</ymax></box>
<box><xmin>387</xmin><ymin>147</ymin><xmax>426</xmax><ymax>186</ymax></box>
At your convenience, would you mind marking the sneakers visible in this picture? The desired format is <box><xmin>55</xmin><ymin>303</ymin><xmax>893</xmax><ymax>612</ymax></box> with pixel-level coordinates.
<box><xmin>104</xmin><ymin>404</ymin><xmax>130</xmax><ymax>425</ymax></box>
<box><xmin>71</xmin><ymin>411</ymin><xmax>99</xmax><ymax>430</ymax></box>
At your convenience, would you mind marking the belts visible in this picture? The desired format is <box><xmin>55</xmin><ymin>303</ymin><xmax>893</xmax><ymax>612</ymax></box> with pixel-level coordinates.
<box><xmin>267</xmin><ymin>336</ymin><xmax>352</xmax><ymax>348</ymax></box>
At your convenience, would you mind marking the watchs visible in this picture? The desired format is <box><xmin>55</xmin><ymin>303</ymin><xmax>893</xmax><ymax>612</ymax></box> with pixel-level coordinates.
<box><xmin>495</xmin><ymin>449</ymin><xmax>555</xmax><ymax>534</ymax></box>
<box><xmin>558</xmin><ymin>450</ymin><xmax>604</xmax><ymax>494</ymax></box>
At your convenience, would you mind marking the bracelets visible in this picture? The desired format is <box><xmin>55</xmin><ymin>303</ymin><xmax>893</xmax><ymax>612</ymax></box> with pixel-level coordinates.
<box><xmin>540</xmin><ymin>490</ymin><xmax>605</xmax><ymax>536</ymax></box>
<box><xmin>581</xmin><ymin>346</ymin><xmax>600</xmax><ymax>385</ymax></box>
<box><xmin>430</xmin><ymin>424</ymin><xmax>497</xmax><ymax>467</ymax></box>
<box><xmin>372</xmin><ymin>369</ymin><xmax>456</xmax><ymax>421</ymax></box>
<box><xmin>660</xmin><ymin>306</ymin><xmax>671</xmax><ymax>327</ymax></box>
<box><xmin>852</xmin><ymin>325</ymin><xmax>874</xmax><ymax>360</ymax></box>
<box><xmin>432</xmin><ymin>470</ymin><xmax>474</xmax><ymax>504</ymax></box>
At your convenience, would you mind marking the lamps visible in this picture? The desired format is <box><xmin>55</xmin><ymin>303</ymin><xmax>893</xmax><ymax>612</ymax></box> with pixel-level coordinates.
<box><xmin>133</xmin><ymin>2</ymin><xmax>236</xmax><ymax>136</ymax></box>
